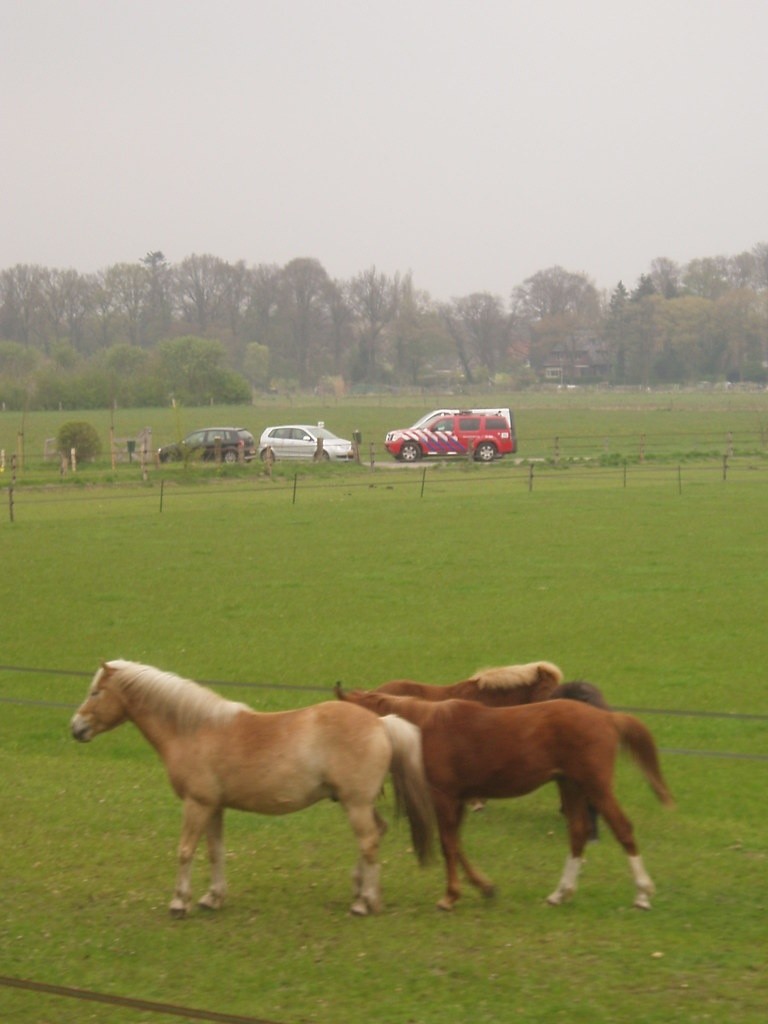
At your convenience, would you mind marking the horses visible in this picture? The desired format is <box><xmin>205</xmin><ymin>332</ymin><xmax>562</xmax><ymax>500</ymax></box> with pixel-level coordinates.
<box><xmin>67</xmin><ymin>659</ymin><xmax>674</xmax><ymax>919</ymax></box>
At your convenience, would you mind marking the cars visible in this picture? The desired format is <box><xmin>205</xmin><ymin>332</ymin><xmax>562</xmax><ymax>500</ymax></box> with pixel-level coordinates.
<box><xmin>156</xmin><ymin>426</ymin><xmax>257</xmax><ymax>465</ymax></box>
<box><xmin>258</xmin><ymin>424</ymin><xmax>354</xmax><ymax>466</ymax></box>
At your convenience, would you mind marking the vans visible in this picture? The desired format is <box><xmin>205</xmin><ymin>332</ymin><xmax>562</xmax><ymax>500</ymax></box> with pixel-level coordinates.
<box><xmin>407</xmin><ymin>409</ymin><xmax>512</xmax><ymax>431</ymax></box>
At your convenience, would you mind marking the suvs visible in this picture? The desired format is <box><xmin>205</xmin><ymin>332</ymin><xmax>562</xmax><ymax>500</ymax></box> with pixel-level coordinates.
<box><xmin>385</xmin><ymin>411</ymin><xmax>514</xmax><ymax>463</ymax></box>
